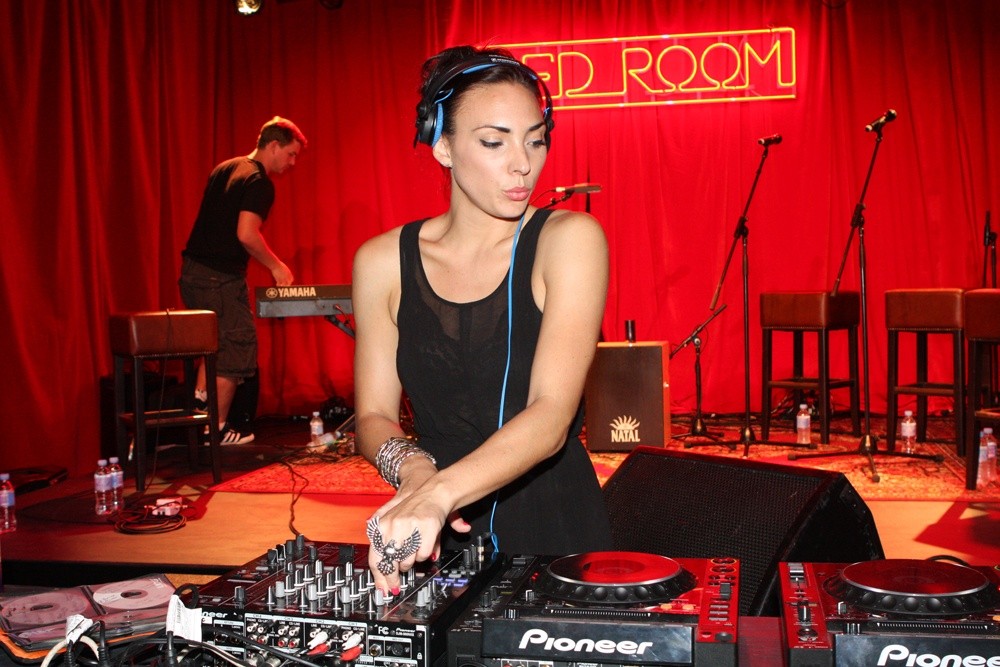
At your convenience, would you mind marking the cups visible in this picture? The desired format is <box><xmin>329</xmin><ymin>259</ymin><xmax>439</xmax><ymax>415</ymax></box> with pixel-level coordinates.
<box><xmin>625</xmin><ymin>320</ymin><xmax>636</xmax><ymax>342</ymax></box>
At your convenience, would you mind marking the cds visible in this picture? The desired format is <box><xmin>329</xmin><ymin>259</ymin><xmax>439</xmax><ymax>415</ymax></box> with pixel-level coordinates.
<box><xmin>93</xmin><ymin>579</ymin><xmax>181</xmax><ymax>637</ymax></box>
<box><xmin>1</xmin><ymin>587</ymin><xmax>90</xmax><ymax>652</ymax></box>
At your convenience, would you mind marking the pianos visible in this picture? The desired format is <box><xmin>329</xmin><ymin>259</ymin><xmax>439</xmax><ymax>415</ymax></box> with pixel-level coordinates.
<box><xmin>251</xmin><ymin>281</ymin><xmax>411</xmax><ymax>448</ymax></box>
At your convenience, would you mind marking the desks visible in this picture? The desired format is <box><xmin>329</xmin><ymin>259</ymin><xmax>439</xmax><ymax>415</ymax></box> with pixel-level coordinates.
<box><xmin>586</xmin><ymin>342</ymin><xmax>669</xmax><ymax>452</ymax></box>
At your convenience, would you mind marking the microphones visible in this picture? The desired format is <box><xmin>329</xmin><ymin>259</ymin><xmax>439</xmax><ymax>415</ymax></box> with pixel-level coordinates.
<box><xmin>553</xmin><ymin>182</ymin><xmax>603</xmax><ymax>195</ymax></box>
<box><xmin>864</xmin><ymin>108</ymin><xmax>899</xmax><ymax>133</ymax></box>
<box><xmin>756</xmin><ymin>133</ymin><xmax>783</xmax><ymax>146</ymax></box>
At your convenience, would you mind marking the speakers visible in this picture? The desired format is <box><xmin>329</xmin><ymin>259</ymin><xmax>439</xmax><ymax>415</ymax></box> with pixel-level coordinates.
<box><xmin>600</xmin><ymin>443</ymin><xmax>885</xmax><ymax>617</ymax></box>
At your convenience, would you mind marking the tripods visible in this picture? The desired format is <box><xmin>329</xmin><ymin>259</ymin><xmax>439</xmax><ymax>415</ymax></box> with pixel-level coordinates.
<box><xmin>788</xmin><ymin>226</ymin><xmax>945</xmax><ymax>483</ymax></box>
<box><xmin>670</xmin><ymin>348</ymin><xmax>725</xmax><ymax>442</ymax></box>
<box><xmin>684</xmin><ymin>236</ymin><xmax>819</xmax><ymax>457</ymax></box>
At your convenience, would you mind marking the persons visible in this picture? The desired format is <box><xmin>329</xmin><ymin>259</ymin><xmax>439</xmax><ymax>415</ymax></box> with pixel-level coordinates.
<box><xmin>351</xmin><ymin>43</ymin><xmax>618</xmax><ymax>607</ymax></box>
<box><xmin>179</xmin><ymin>114</ymin><xmax>308</xmax><ymax>447</ymax></box>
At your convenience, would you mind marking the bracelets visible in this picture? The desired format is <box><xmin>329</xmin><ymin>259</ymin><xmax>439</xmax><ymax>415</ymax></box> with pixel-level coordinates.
<box><xmin>374</xmin><ymin>436</ymin><xmax>437</xmax><ymax>490</ymax></box>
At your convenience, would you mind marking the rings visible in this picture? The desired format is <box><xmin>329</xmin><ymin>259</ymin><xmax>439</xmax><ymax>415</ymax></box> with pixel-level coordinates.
<box><xmin>366</xmin><ymin>515</ymin><xmax>423</xmax><ymax>576</ymax></box>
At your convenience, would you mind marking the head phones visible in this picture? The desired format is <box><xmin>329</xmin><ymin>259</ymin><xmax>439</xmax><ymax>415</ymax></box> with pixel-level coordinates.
<box><xmin>412</xmin><ymin>54</ymin><xmax>555</xmax><ymax>148</ymax></box>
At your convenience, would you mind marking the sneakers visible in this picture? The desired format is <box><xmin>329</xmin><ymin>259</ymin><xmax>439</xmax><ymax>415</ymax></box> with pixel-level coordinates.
<box><xmin>193</xmin><ymin>389</ymin><xmax>210</xmax><ymax>414</ymax></box>
<box><xmin>204</xmin><ymin>422</ymin><xmax>255</xmax><ymax>445</ymax></box>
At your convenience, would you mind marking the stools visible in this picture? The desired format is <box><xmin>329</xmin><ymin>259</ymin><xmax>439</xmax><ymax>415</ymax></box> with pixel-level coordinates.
<box><xmin>885</xmin><ymin>288</ymin><xmax>968</xmax><ymax>454</ymax></box>
<box><xmin>108</xmin><ymin>310</ymin><xmax>221</xmax><ymax>495</ymax></box>
<box><xmin>759</xmin><ymin>291</ymin><xmax>863</xmax><ymax>443</ymax></box>
<box><xmin>965</xmin><ymin>287</ymin><xmax>1000</xmax><ymax>490</ymax></box>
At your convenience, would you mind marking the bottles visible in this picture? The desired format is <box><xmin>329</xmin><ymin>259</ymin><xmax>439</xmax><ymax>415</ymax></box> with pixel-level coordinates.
<box><xmin>311</xmin><ymin>411</ymin><xmax>324</xmax><ymax>442</ymax></box>
<box><xmin>0</xmin><ymin>474</ymin><xmax>17</xmax><ymax>533</ymax></box>
<box><xmin>982</xmin><ymin>428</ymin><xmax>997</xmax><ymax>481</ymax></box>
<box><xmin>900</xmin><ymin>410</ymin><xmax>917</xmax><ymax>454</ymax></box>
<box><xmin>976</xmin><ymin>432</ymin><xmax>990</xmax><ymax>488</ymax></box>
<box><xmin>306</xmin><ymin>431</ymin><xmax>341</xmax><ymax>454</ymax></box>
<box><xmin>94</xmin><ymin>459</ymin><xmax>113</xmax><ymax>515</ymax></box>
<box><xmin>107</xmin><ymin>457</ymin><xmax>124</xmax><ymax>510</ymax></box>
<box><xmin>796</xmin><ymin>403</ymin><xmax>811</xmax><ymax>444</ymax></box>
